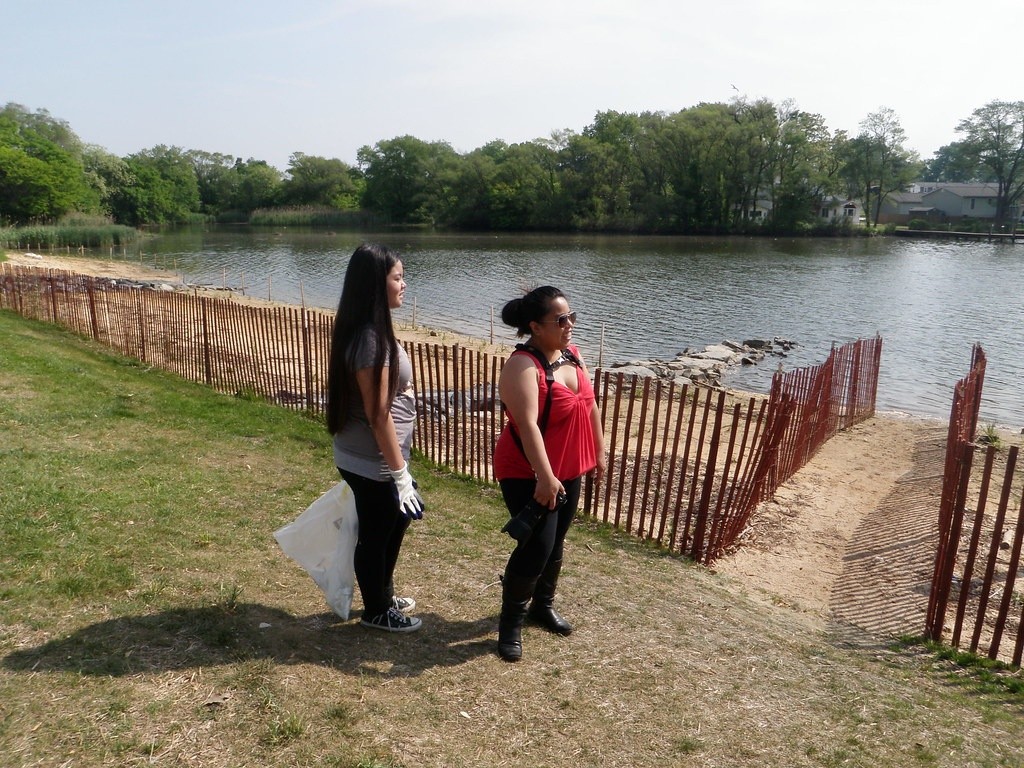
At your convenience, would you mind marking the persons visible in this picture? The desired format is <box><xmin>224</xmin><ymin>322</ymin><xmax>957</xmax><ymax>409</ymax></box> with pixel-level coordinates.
<box><xmin>326</xmin><ymin>243</ymin><xmax>427</xmax><ymax>633</ymax></box>
<box><xmin>493</xmin><ymin>286</ymin><xmax>606</xmax><ymax>660</ymax></box>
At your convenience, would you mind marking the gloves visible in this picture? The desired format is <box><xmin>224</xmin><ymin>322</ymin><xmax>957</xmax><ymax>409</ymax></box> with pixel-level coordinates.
<box><xmin>388</xmin><ymin>460</ymin><xmax>425</xmax><ymax>520</ymax></box>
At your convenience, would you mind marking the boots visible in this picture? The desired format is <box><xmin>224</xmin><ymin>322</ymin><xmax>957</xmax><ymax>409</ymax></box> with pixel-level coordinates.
<box><xmin>527</xmin><ymin>557</ymin><xmax>573</xmax><ymax>636</ymax></box>
<box><xmin>497</xmin><ymin>556</ymin><xmax>541</xmax><ymax>660</ymax></box>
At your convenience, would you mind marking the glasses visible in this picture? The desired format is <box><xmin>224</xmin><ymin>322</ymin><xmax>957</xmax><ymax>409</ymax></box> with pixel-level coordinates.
<box><xmin>539</xmin><ymin>311</ymin><xmax>576</xmax><ymax>328</ymax></box>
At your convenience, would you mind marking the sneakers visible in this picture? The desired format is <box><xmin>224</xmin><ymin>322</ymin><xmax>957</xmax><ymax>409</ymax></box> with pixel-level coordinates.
<box><xmin>361</xmin><ymin>607</ymin><xmax>423</xmax><ymax>632</ymax></box>
<box><xmin>392</xmin><ymin>596</ymin><xmax>416</xmax><ymax>612</ymax></box>
